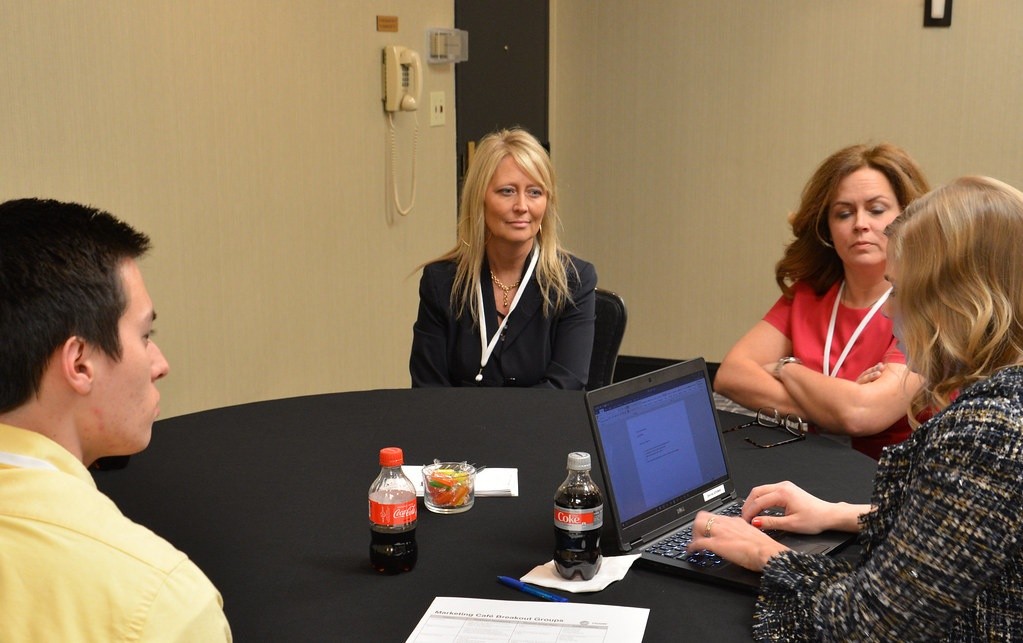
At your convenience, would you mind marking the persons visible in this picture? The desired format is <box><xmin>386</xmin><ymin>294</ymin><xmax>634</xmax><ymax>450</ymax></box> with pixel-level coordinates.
<box><xmin>686</xmin><ymin>174</ymin><xmax>1023</xmax><ymax>643</ymax></box>
<box><xmin>714</xmin><ymin>143</ymin><xmax>960</xmax><ymax>457</ymax></box>
<box><xmin>410</xmin><ymin>129</ymin><xmax>597</xmax><ymax>391</ymax></box>
<box><xmin>0</xmin><ymin>198</ymin><xmax>233</xmax><ymax>643</ymax></box>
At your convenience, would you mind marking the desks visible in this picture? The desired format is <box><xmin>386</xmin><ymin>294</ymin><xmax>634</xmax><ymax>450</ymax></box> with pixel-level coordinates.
<box><xmin>87</xmin><ymin>388</ymin><xmax>880</xmax><ymax>643</ymax></box>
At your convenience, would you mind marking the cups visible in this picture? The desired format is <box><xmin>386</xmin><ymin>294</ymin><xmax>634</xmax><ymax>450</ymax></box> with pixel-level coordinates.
<box><xmin>423</xmin><ymin>463</ymin><xmax>477</xmax><ymax>514</ymax></box>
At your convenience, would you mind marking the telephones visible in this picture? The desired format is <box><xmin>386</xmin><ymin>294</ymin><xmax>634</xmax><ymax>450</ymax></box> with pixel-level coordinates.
<box><xmin>383</xmin><ymin>46</ymin><xmax>424</xmax><ymax>112</ymax></box>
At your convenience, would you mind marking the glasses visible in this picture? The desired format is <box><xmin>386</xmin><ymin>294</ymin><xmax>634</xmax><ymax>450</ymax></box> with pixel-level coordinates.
<box><xmin>721</xmin><ymin>406</ymin><xmax>806</xmax><ymax>448</ymax></box>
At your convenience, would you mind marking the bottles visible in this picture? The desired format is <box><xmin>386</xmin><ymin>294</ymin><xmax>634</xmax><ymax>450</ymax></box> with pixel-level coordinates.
<box><xmin>368</xmin><ymin>447</ymin><xmax>418</xmax><ymax>575</ymax></box>
<box><xmin>552</xmin><ymin>452</ymin><xmax>604</xmax><ymax>581</ymax></box>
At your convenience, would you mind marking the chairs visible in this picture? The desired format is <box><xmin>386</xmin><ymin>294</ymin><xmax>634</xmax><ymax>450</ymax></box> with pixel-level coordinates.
<box><xmin>586</xmin><ymin>288</ymin><xmax>627</xmax><ymax>389</ymax></box>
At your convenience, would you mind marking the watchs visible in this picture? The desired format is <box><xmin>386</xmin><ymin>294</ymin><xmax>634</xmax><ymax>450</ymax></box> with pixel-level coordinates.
<box><xmin>773</xmin><ymin>357</ymin><xmax>800</xmax><ymax>378</ymax></box>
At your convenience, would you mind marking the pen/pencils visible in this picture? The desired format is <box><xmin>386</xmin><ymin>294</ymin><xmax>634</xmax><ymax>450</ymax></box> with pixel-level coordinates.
<box><xmin>498</xmin><ymin>575</ymin><xmax>569</xmax><ymax>602</ymax></box>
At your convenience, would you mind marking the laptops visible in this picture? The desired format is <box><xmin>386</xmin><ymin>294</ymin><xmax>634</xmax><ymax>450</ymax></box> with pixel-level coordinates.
<box><xmin>584</xmin><ymin>356</ymin><xmax>857</xmax><ymax>593</ymax></box>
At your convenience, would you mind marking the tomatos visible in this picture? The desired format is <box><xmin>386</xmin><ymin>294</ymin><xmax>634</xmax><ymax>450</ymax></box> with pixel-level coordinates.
<box><xmin>425</xmin><ymin>473</ymin><xmax>469</xmax><ymax>506</ymax></box>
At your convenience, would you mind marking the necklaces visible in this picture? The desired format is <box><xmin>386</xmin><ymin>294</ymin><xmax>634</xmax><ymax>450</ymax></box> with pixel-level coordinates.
<box><xmin>490</xmin><ymin>270</ymin><xmax>520</xmax><ymax>305</ymax></box>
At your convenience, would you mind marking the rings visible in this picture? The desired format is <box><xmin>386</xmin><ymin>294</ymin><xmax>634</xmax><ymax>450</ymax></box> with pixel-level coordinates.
<box><xmin>704</xmin><ymin>518</ymin><xmax>716</xmax><ymax>537</ymax></box>
